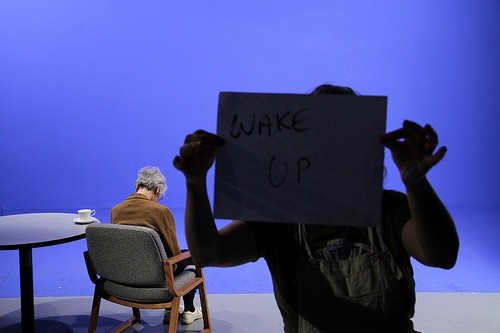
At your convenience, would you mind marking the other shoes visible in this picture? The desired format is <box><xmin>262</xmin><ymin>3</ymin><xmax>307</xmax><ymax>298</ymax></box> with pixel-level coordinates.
<box><xmin>181</xmin><ymin>307</ymin><xmax>203</xmax><ymax>324</ymax></box>
<box><xmin>163</xmin><ymin>305</ymin><xmax>185</xmax><ymax>324</ymax></box>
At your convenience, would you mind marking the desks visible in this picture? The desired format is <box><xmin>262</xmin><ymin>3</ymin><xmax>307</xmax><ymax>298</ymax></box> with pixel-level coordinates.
<box><xmin>0</xmin><ymin>212</ymin><xmax>100</xmax><ymax>333</ymax></box>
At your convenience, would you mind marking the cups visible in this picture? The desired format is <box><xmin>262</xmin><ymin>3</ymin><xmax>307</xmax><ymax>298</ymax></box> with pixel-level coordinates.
<box><xmin>78</xmin><ymin>209</ymin><xmax>95</xmax><ymax>220</ymax></box>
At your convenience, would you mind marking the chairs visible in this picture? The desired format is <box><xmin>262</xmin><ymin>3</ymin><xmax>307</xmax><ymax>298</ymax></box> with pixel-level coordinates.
<box><xmin>82</xmin><ymin>224</ymin><xmax>212</xmax><ymax>333</ymax></box>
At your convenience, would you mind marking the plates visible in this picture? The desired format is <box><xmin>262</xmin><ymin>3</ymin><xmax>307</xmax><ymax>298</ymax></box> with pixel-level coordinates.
<box><xmin>73</xmin><ymin>218</ymin><xmax>94</xmax><ymax>223</ymax></box>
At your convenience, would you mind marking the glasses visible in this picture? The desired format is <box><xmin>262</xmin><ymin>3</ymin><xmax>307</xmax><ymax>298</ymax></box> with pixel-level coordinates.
<box><xmin>159</xmin><ymin>193</ymin><xmax>163</xmax><ymax>200</ymax></box>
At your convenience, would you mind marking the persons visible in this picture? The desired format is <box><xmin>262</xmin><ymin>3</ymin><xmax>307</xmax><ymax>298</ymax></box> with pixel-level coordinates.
<box><xmin>173</xmin><ymin>82</ymin><xmax>459</xmax><ymax>333</ymax></box>
<box><xmin>109</xmin><ymin>165</ymin><xmax>206</xmax><ymax>328</ymax></box>
